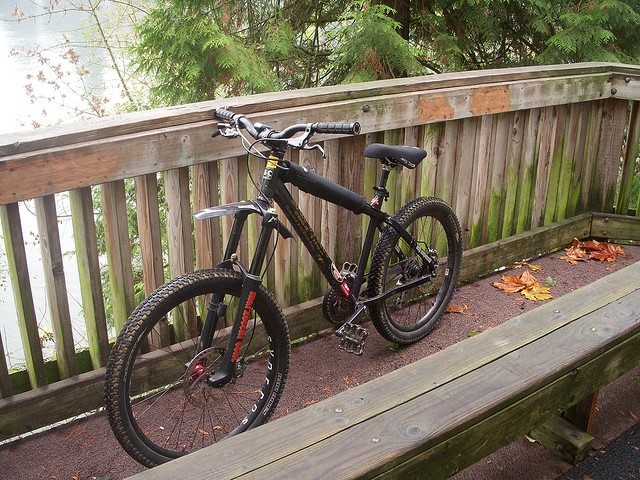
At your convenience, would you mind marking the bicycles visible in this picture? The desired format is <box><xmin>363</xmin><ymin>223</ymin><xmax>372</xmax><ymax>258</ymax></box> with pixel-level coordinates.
<box><xmin>104</xmin><ymin>108</ymin><xmax>464</xmax><ymax>467</ymax></box>
